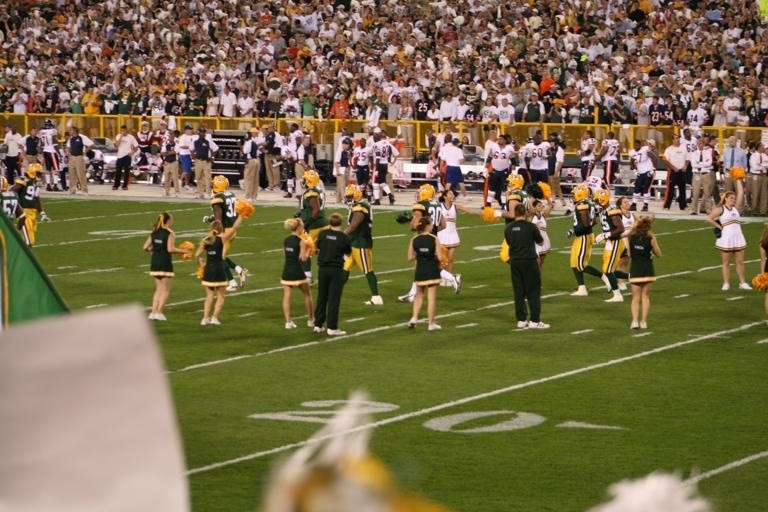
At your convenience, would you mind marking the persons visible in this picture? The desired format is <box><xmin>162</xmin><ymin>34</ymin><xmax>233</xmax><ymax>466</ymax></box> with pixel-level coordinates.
<box><xmin>295</xmin><ymin>169</ymin><xmax>332</xmax><ymax>277</ymax></box>
<box><xmin>279</xmin><ymin>217</ymin><xmax>314</xmax><ymax>330</ymax></box>
<box><xmin>708</xmin><ymin>192</ymin><xmax>752</xmax><ymax>290</ymax></box>
<box><xmin>403</xmin><ymin>217</ymin><xmax>446</xmax><ymax>332</ymax></box>
<box><xmin>484</xmin><ymin>174</ymin><xmax>553</xmax><ymax>266</ymax></box>
<box><xmin>312</xmin><ymin>213</ymin><xmax>352</xmax><ymax>338</ymax></box>
<box><xmin>567</xmin><ymin>185</ymin><xmax>636</xmax><ymax>302</ymax></box>
<box><xmin>342</xmin><ymin>184</ymin><xmax>383</xmax><ymax>305</ymax></box>
<box><xmin>500</xmin><ymin>205</ymin><xmax>550</xmax><ymax>331</ymax></box>
<box><xmin>1</xmin><ymin>164</ymin><xmax>52</xmax><ymax>246</ymax></box>
<box><xmin>203</xmin><ymin>176</ymin><xmax>248</xmax><ymax>293</ymax></box>
<box><xmin>626</xmin><ymin>216</ymin><xmax>662</xmax><ymax>331</ymax></box>
<box><xmin>1</xmin><ymin>1</ymin><xmax>767</xmax><ymax>216</ymax></box>
<box><xmin>143</xmin><ymin>211</ymin><xmax>189</xmax><ymax>321</ymax></box>
<box><xmin>193</xmin><ymin>209</ymin><xmax>248</xmax><ymax>326</ymax></box>
<box><xmin>757</xmin><ymin>217</ymin><xmax>767</xmax><ymax>320</ymax></box>
<box><xmin>399</xmin><ymin>185</ymin><xmax>462</xmax><ymax>302</ymax></box>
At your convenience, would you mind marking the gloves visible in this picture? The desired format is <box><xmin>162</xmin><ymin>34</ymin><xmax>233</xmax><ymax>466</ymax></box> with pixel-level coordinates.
<box><xmin>566</xmin><ymin>229</ymin><xmax>575</xmax><ymax>239</ymax></box>
<box><xmin>595</xmin><ymin>233</ymin><xmax>608</xmax><ymax>244</ymax></box>
<box><xmin>203</xmin><ymin>215</ymin><xmax>214</xmax><ymax>224</ymax></box>
<box><xmin>40</xmin><ymin>214</ymin><xmax>50</xmax><ymax>222</ymax></box>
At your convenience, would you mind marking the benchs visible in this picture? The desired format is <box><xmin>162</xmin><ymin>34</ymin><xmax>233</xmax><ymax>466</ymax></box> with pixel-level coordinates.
<box><xmin>394</xmin><ymin>157</ymin><xmax>674</xmax><ymax>201</ymax></box>
<box><xmin>100</xmin><ymin>153</ymin><xmax>163</xmax><ymax>184</ymax></box>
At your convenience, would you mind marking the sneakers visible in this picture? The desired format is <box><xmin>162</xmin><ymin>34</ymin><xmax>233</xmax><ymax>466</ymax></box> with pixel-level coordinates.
<box><xmin>527</xmin><ymin>321</ymin><xmax>550</xmax><ymax>329</ymax></box>
<box><xmin>153</xmin><ymin>313</ymin><xmax>167</xmax><ymax>321</ymax></box>
<box><xmin>239</xmin><ymin>268</ymin><xmax>249</xmax><ymax>289</ymax></box>
<box><xmin>148</xmin><ymin>313</ymin><xmax>153</xmax><ymax>319</ymax></box>
<box><xmin>630</xmin><ymin>321</ymin><xmax>638</xmax><ymax>329</ymax></box>
<box><xmin>226</xmin><ymin>285</ymin><xmax>240</xmax><ymax>292</ymax></box>
<box><xmin>738</xmin><ymin>283</ymin><xmax>753</xmax><ymax>290</ymax></box>
<box><xmin>570</xmin><ymin>282</ymin><xmax>628</xmax><ymax>302</ymax></box>
<box><xmin>285</xmin><ymin>318</ymin><xmax>347</xmax><ymax>335</ymax></box>
<box><xmin>721</xmin><ymin>284</ymin><xmax>729</xmax><ymax>291</ymax></box>
<box><xmin>361</xmin><ymin>292</ymin><xmax>441</xmax><ymax>332</ymax></box>
<box><xmin>209</xmin><ymin>318</ymin><xmax>221</xmax><ymax>325</ymax></box>
<box><xmin>439</xmin><ymin>273</ymin><xmax>462</xmax><ymax>294</ymax></box>
<box><xmin>517</xmin><ymin>320</ymin><xmax>528</xmax><ymax>328</ymax></box>
<box><xmin>200</xmin><ymin>319</ymin><xmax>209</xmax><ymax>326</ymax></box>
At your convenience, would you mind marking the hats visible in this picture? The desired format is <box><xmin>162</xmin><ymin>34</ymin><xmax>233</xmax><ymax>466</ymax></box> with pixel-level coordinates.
<box><xmin>646</xmin><ymin>139</ymin><xmax>654</xmax><ymax>146</ymax></box>
<box><xmin>185</xmin><ymin>124</ymin><xmax>192</xmax><ymax>129</ymax></box>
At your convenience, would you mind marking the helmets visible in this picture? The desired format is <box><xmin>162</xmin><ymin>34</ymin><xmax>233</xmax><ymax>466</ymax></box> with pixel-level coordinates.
<box><xmin>26</xmin><ymin>162</ymin><xmax>42</xmax><ymax>180</ymax></box>
<box><xmin>299</xmin><ymin>170</ymin><xmax>320</xmax><ymax>188</ymax></box>
<box><xmin>343</xmin><ymin>184</ymin><xmax>363</xmax><ymax>207</ymax></box>
<box><xmin>415</xmin><ymin>183</ymin><xmax>435</xmax><ymax>202</ymax></box>
<box><xmin>211</xmin><ymin>175</ymin><xmax>230</xmax><ymax>193</ymax></box>
<box><xmin>570</xmin><ymin>185</ymin><xmax>590</xmax><ymax>205</ymax></box>
<box><xmin>0</xmin><ymin>177</ymin><xmax>9</xmax><ymax>191</ymax></box>
<box><xmin>591</xmin><ymin>189</ymin><xmax>610</xmax><ymax>208</ymax></box>
<box><xmin>505</xmin><ymin>174</ymin><xmax>525</xmax><ymax>192</ymax></box>
<box><xmin>42</xmin><ymin>119</ymin><xmax>54</xmax><ymax>128</ymax></box>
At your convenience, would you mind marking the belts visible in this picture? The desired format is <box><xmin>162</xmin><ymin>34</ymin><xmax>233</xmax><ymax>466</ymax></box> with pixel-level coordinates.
<box><xmin>695</xmin><ymin>172</ymin><xmax>707</xmax><ymax>174</ymax></box>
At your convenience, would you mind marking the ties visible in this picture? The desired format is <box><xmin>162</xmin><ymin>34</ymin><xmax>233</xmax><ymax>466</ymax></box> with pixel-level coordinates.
<box><xmin>730</xmin><ymin>149</ymin><xmax>733</xmax><ymax>168</ymax></box>
<box><xmin>698</xmin><ymin>153</ymin><xmax>701</xmax><ymax>171</ymax></box>
<box><xmin>759</xmin><ymin>154</ymin><xmax>762</xmax><ymax>176</ymax></box>
